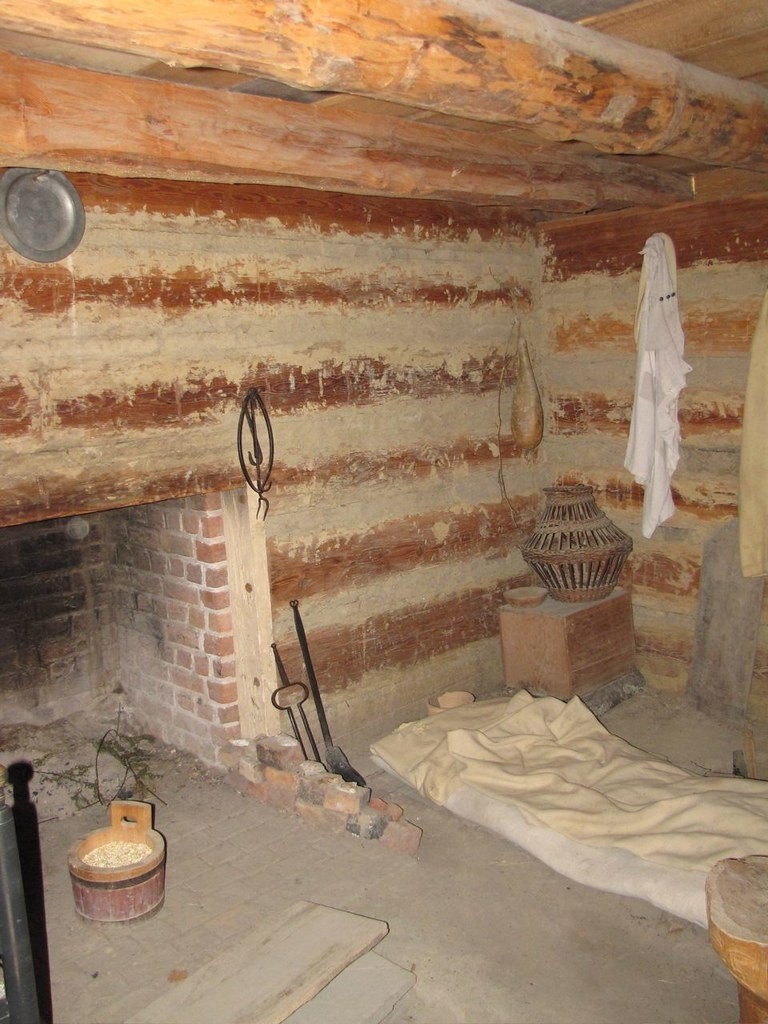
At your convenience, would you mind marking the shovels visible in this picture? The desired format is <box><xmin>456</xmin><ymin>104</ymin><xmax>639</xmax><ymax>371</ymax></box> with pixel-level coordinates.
<box><xmin>289</xmin><ymin>598</ymin><xmax>367</xmax><ymax>788</ymax></box>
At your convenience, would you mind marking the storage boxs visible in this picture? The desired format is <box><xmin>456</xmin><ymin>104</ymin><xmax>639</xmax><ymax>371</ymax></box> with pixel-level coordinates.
<box><xmin>496</xmin><ymin>584</ymin><xmax>639</xmax><ymax>700</ymax></box>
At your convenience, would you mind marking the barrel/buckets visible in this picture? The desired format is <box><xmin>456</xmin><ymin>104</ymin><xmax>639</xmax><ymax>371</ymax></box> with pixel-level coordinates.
<box><xmin>66</xmin><ymin>799</ymin><xmax>165</xmax><ymax>928</ymax></box>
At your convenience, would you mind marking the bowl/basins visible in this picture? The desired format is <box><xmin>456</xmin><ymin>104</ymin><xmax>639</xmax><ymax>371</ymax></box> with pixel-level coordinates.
<box><xmin>503</xmin><ymin>585</ymin><xmax>549</xmax><ymax>609</ymax></box>
<box><xmin>427</xmin><ymin>690</ymin><xmax>475</xmax><ymax>715</ymax></box>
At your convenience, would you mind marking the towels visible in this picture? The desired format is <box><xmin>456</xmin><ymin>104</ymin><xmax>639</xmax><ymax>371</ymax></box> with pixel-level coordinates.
<box><xmin>621</xmin><ymin>230</ymin><xmax>694</xmax><ymax>539</ymax></box>
